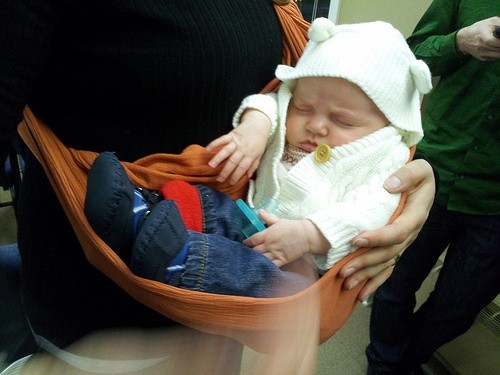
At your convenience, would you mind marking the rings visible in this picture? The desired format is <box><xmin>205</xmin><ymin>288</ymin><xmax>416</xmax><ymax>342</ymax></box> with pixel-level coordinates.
<box><xmin>394</xmin><ymin>254</ymin><xmax>401</xmax><ymax>265</ymax></box>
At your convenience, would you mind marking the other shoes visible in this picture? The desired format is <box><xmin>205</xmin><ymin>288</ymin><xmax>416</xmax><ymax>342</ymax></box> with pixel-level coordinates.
<box><xmin>128</xmin><ymin>201</ymin><xmax>188</xmax><ymax>282</ymax></box>
<box><xmin>416</xmin><ymin>356</ymin><xmax>451</xmax><ymax>375</ymax></box>
<box><xmin>85</xmin><ymin>151</ymin><xmax>134</xmax><ymax>248</ymax></box>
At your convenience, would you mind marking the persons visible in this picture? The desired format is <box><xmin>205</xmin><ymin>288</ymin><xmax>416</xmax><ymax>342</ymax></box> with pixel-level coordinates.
<box><xmin>0</xmin><ymin>0</ymin><xmax>436</xmax><ymax>375</ymax></box>
<box><xmin>365</xmin><ymin>1</ymin><xmax>500</xmax><ymax>375</ymax></box>
<box><xmin>83</xmin><ymin>17</ymin><xmax>433</xmax><ymax>352</ymax></box>
